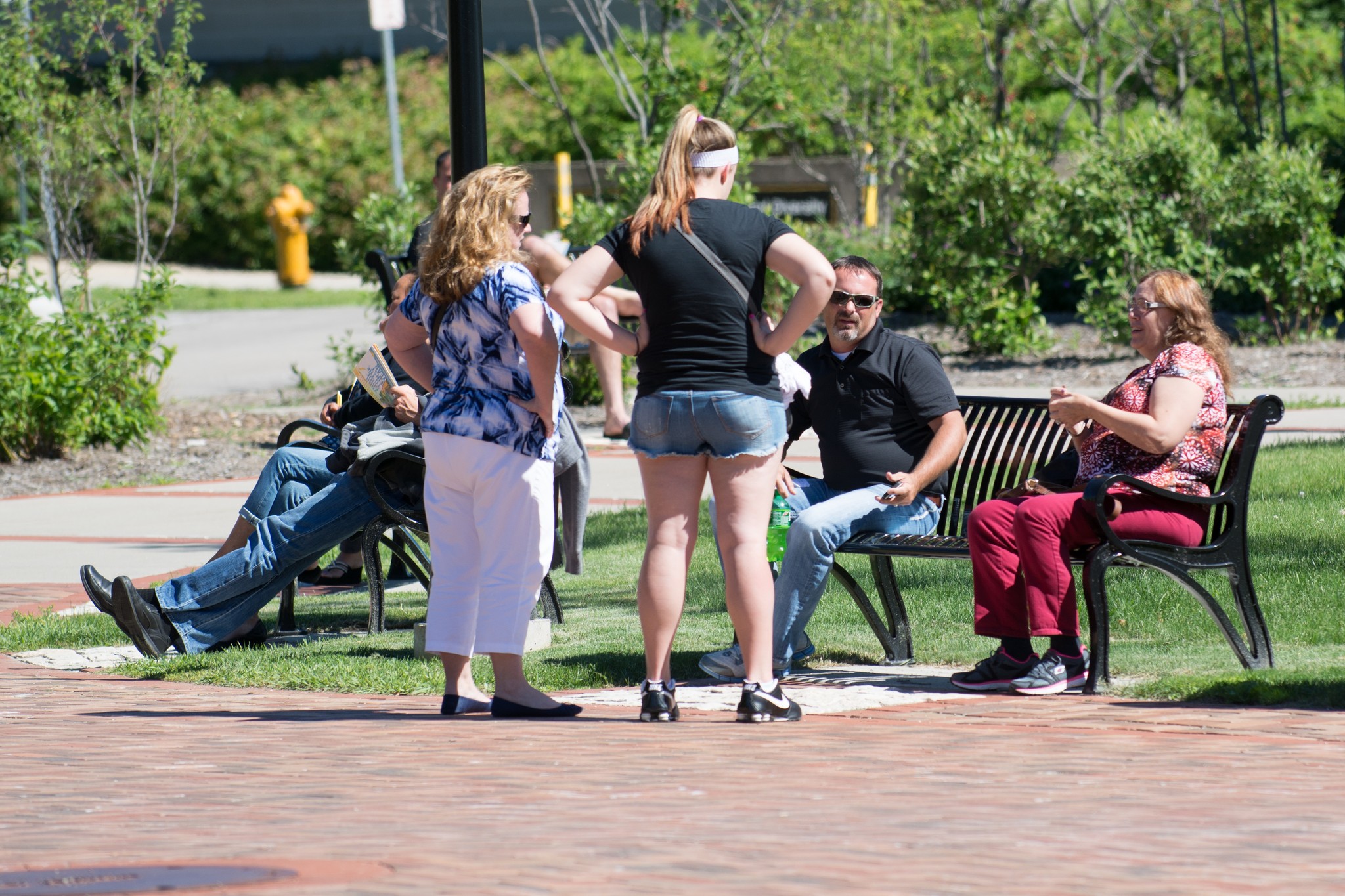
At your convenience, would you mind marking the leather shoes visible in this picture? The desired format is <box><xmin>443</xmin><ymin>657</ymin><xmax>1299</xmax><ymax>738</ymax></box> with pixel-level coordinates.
<box><xmin>111</xmin><ymin>575</ymin><xmax>172</xmax><ymax>660</ymax></box>
<box><xmin>80</xmin><ymin>565</ymin><xmax>130</xmax><ymax>637</ymax></box>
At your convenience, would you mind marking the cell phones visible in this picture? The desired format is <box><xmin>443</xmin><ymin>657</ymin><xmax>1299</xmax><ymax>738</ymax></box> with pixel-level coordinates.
<box><xmin>882</xmin><ymin>482</ymin><xmax>903</xmax><ymax>499</ymax></box>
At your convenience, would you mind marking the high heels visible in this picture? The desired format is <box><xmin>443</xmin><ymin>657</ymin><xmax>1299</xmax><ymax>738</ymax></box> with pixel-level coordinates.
<box><xmin>314</xmin><ymin>559</ymin><xmax>363</xmax><ymax>585</ymax></box>
<box><xmin>298</xmin><ymin>565</ymin><xmax>322</xmax><ymax>583</ymax></box>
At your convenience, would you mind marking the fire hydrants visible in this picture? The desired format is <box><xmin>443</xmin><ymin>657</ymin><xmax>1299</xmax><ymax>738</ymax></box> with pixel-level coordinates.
<box><xmin>268</xmin><ymin>183</ymin><xmax>319</xmax><ymax>295</ymax></box>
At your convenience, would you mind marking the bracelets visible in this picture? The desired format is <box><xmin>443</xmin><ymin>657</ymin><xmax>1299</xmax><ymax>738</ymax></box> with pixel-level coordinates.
<box><xmin>1066</xmin><ymin>422</ymin><xmax>1085</xmax><ymax>437</ymax></box>
<box><xmin>634</xmin><ymin>334</ymin><xmax>639</xmax><ymax>358</ymax></box>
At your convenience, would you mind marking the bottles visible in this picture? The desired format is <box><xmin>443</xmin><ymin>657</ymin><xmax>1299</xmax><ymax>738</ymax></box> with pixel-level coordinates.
<box><xmin>766</xmin><ymin>489</ymin><xmax>791</xmax><ymax>562</ymax></box>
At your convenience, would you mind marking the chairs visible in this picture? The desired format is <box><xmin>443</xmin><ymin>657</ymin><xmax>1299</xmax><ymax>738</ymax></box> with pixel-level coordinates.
<box><xmin>272</xmin><ymin>247</ymin><xmax>575</xmax><ymax>638</ymax></box>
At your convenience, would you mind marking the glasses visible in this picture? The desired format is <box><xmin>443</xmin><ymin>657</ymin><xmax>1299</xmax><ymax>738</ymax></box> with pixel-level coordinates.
<box><xmin>1127</xmin><ymin>300</ymin><xmax>1167</xmax><ymax>311</ymax></box>
<box><xmin>510</xmin><ymin>213</ymin><xmax>531</xmax><ymax>229</ymax></box>
<box><xmin>829</xmin><ymin>291</ymin><xmax>878</xmax><ymax>308</ymax></box>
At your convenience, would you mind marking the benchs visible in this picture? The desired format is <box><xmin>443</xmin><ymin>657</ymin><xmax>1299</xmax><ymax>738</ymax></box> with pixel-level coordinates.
<box><xmin>823</xmin><ymin>393</ymin><xmax>1288</xmax><ymax>695</ymax></box>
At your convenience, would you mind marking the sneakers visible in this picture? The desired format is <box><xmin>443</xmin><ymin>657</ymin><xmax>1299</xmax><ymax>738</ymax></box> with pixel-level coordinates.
<box><xmin>639</xmin><ymin>679</ymin><xmax>679</xmax><ymax>722</ymax></box>
<box><xmin>698</xmin><ymin>644</ymin><xmax>792</xmax><ymax>683</ymax></box>
<box><xmin>950</xmin><ymin>646</ymin><xmax>1039</xmax><ymax>691</ymax></box>
<box><xmin>733</xmin><ymin>678</ymin><xmax>802</xmax><ymax>722</ymax></box>
<box><xmin>1009</xmin><ymin>644</ymin><xmax>1090</xmax><ymax>695</ymax></box>
<box><xmin>791</xmin><ymin>631</ymin><xmax>816</xmax><ymax>660</ymax></box>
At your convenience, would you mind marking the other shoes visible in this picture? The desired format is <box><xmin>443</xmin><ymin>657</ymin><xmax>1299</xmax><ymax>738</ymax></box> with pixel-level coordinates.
<box><xmin>207</xmin><ymin>619</ymin><xmax>267</xmax><ymax>653</ymax></box>
<box><xmin>440</xmin><ymin>694</ymin><xmax>493</xmax><ymax>714</ymax></box>
<box><xmin>491</xmin><ymin>695</ymin><xmax>582</xmax><ymax>718</ymax></box>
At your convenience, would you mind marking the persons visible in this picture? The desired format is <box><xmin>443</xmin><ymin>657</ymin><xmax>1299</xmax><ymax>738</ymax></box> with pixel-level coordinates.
<box><xmin>386</xmin><ymin>162</ymin><xmax>583</xmax><ymax>720</ymax></box>
<box><xmin>297</xmin><ymin>271</ymin><xmax>420</xmax><ymax>585</ymax></box>
<box><xmin>950</xmin><ymin>269</ymin><xmax>1234</xmax><ymax>695</ymax></box>
<box><xmin>545</xmin><ymin>102</ymin><xmax>838</xmax><ymax>722</ymax></box>
<box><xmin>699</xmin><ymin>255</ymin><xmax>967</xmax><ymax>683</ymax></box>
<box><xmin>408</xmin><ymin>151</ymin><xmax>453</xmax><ymax>267</ymax></box>
<box><xmin>80</xmin><ymin>385</ymin><xmax>431</xmax><ymax>660</ymax></box>
<box><xmin>520</xmin><ymin>235</ymin><xmax>643</xmax><ymax>440</ymax></box>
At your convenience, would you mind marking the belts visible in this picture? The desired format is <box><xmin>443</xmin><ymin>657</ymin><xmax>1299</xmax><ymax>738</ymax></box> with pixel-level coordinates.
<box><xmin>920</xmin><ymin>490</ymin><xmax>941</xmax><ymax>509</ymax></box>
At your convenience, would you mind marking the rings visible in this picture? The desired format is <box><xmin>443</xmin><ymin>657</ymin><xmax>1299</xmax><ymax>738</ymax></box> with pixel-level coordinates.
<box><xmin>1057</xmin><ymin>419</ymin><xmax>1062</xmax><ymax>425</ymax></box>
